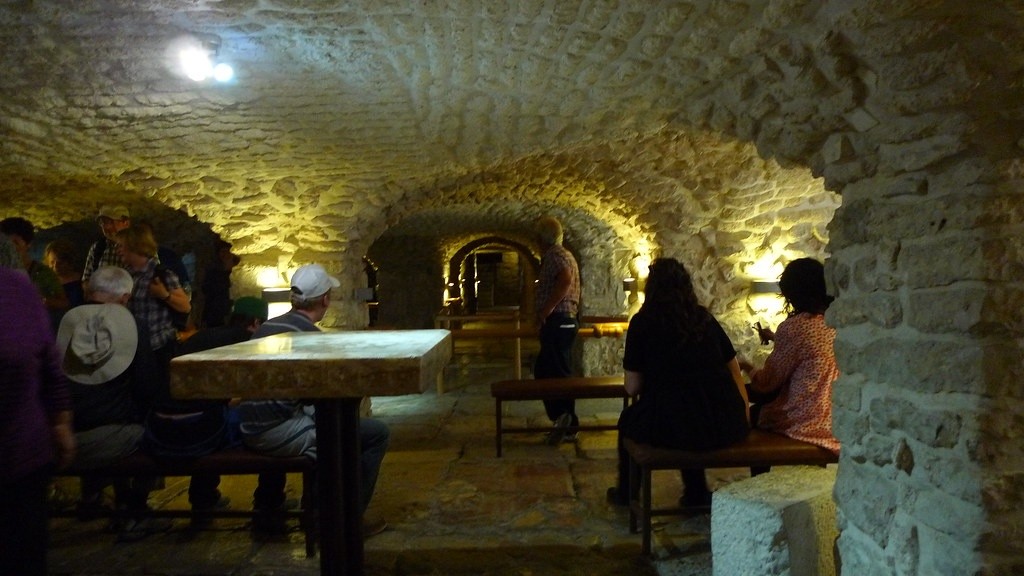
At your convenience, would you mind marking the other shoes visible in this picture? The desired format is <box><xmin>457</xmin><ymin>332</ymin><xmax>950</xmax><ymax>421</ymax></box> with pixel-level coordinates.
<box><xmin>544</xmin><ymin>424</ymin><xmax>580</xmax><ymax>442</ymax></box>
<box><xmin>607</xmin><ymin>487</ymin><xmax>625</xmax><ymax>505</ymax></box>
<box><xmin>679</xmin><ymin>495</ymin><xmax>711</xmax><ymax>510</ymax></box>
<box><xmin>544</xmin><ymin>414</ymin><xmax>572</xmax><ymax>447</ymax></box>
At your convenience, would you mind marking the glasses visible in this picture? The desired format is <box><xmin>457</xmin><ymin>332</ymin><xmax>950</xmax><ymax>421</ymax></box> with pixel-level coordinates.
<box><xmin>99</xmin><ymin>218</ymin><xmax>125</xmax><ymax>228</ymax></box>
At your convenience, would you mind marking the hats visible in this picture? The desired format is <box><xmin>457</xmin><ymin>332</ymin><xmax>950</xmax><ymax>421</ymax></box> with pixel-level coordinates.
<box><xmin>233</xmin><ymin>296</ymin><xmax>268</xmax><ymax>320</ymax></box>
<box><xmin>290</xmin><ymin>265</ymin><xmax>341</xmax><ymax>299</ymax></box>
<box><xmin>94</xmin><ymin>202</ymin><xmax>130</xmax><ymax>220</ymax></box>
<box><xmin>55</xmin><ymin>302</ymin><xmax>138</xmax><ymax>386</ymax></box>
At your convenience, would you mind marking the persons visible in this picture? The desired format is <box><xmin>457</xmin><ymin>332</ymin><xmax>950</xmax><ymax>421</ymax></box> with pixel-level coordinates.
<box><xmin>738</xmin><ymin>258</ymin><xmax>841</xmax><ymax>477</ymax></box>
<box><xmin>240</xmin><ymin>265</ymin><xmax>390</xmax><ymax>540</ymax></box>
<box><xmin>607</xmin><ymin>259</ymin><xmax>750</xmax><ymax>516</ymax></box>
<box><xmin>0</xmin><ymin>205</ymin><xmax>299</xmax><ymax>576</ymax></box>
<box><xmin>528</xmin><ymin>217</ymin><xmax>581</xmax><ymax>444</ymax></box>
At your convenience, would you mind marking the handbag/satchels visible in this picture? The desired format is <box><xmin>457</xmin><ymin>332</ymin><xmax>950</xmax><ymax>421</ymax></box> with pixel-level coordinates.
<box><xmin>545</xmin><ymin>318</ymin><xmax>579</xmax><ymax>341</ymax></box>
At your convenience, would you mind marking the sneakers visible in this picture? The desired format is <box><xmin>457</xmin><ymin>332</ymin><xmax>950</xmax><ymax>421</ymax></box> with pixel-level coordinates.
<box><xmin>251</xmin><ymin>498</ymin><xmax>299</xmax><ymax>517</ymax></box>
<box><xmin>117</xmin><ymin>505</ymin><xmax>177</xmax><ymax>542</ymax></box>
<box><xmin>76</xmin><ymin>492</ymin><xmax>117</xmax><ymax>522</ymax></box>
<box><xmin>363</xmin><ymin>518</ymin><xmax>387</xmax><ymax>537</ymax></box>
<box><xmin>190</xmin><ymin>496</ymin><xmax>231</xmax><ymax>512</ymax></box>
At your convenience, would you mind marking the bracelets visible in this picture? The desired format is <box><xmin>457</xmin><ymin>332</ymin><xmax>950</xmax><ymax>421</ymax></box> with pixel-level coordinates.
<box><xmin>163</xmin><ymin>294</ymin><xmax>171</xmax><ymax>301</ymax></box>
<box><xmin>745</xmin><ymin>404</ymin><xmax>749</xmax><ymax>408</ymax></box>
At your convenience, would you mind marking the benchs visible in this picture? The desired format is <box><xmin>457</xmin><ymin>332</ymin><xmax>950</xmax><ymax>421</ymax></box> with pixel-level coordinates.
<box><xmin>621</xmin><ymin>430</ymin><xmax>840</xmax><ymax>554</ymax></box>
<box><xmin>490</xmin><ymin>374</ymin><xmax>634</xmax><ymax>458</ymax></box>
<box><xmin>51</xmin><ymin>453</ymin><xmax>317</xmax><ymax>559</ymax></box>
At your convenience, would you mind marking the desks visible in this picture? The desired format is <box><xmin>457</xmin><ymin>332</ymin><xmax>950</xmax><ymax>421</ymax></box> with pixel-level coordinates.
<box><xmin>174</xmin><ymin>329</ymin><xmax>453</xmax><ymax>576</ymax></box>
